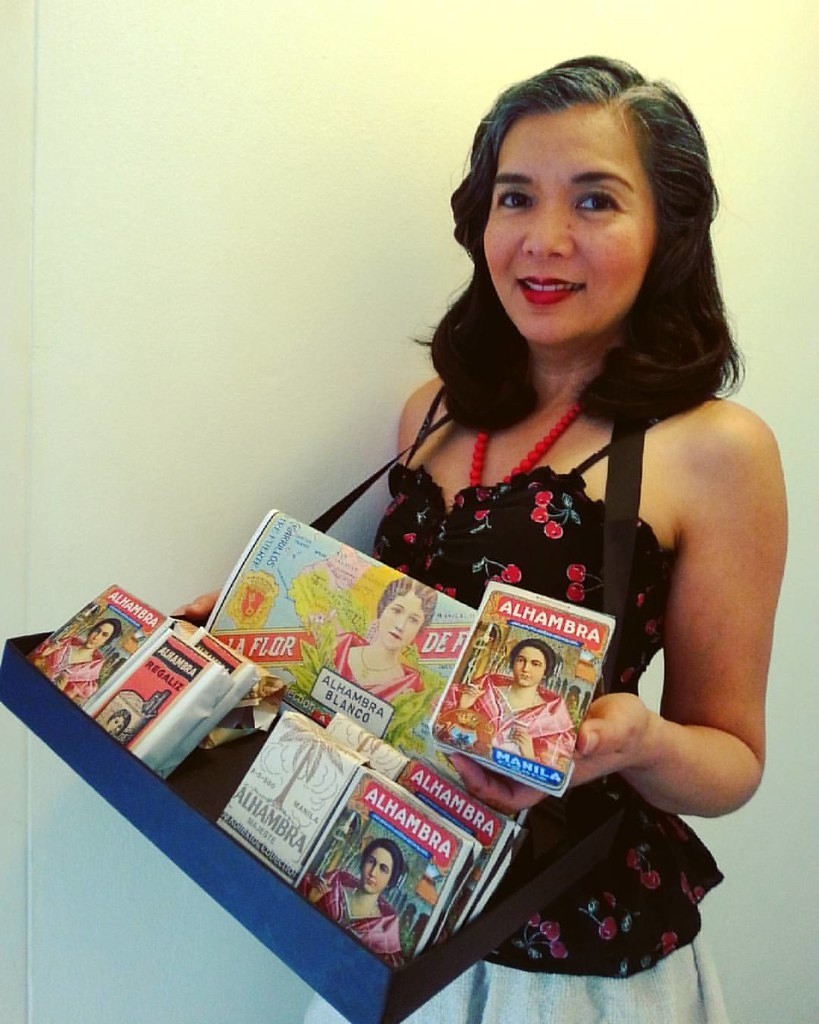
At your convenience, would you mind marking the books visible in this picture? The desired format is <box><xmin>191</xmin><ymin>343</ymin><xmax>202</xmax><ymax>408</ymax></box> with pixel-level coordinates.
<box><xmin>326</xmin><ymin>713</ymin><xmax>408</xmax><ymax>781</ymax></box>
<box><xmin>27</xmin><ymin>583</ymin><xmax>173</xmax><ymax>710</ymax></box>
<box><xmin>86</xmin><ymin>630</ymin><xmax>234</xmax><ymax>772</ymax></box>
<box><xmin>152</xmin><ymin>628</ymin><xmax>261</xmax><ymax>779</ymax></box>
<box><xmin>395</xmin><ymin>760</ymin><xmax>522</xmax><ymax>944</ymax></box>
<box><xmin>431</xmin><ymin>580</ymin><xmax>614</xmax><ymax>798</ymax></box>
<box><xmin>216</xmin><ymin>707</ymin><xmax>373</xmax><ymax>889</ymax></box>
<box><xmin>294</xmin><ymin>766</ymin><xmax>482</xmax><ymax>969</ymax></box>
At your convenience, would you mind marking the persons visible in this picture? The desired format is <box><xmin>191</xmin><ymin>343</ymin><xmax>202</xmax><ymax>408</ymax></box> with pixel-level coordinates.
<box><xmin>306</xmin><ymin>55</ymin><xmax>789</xmax><ymax>1024</ymax></box>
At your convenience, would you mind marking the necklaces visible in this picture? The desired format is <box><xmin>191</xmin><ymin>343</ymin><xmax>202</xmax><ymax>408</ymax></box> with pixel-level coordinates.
<box><xmin>470</xmin><ymin>407</ymin><xmax>580</xmax><ymax>486</ymax></box>
